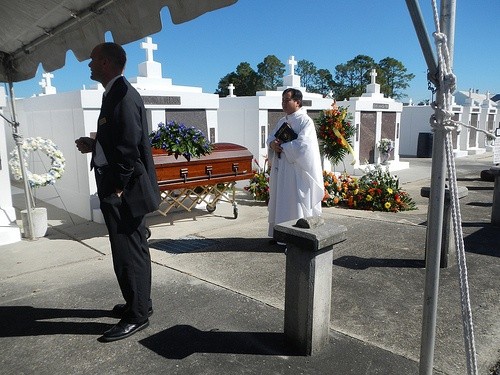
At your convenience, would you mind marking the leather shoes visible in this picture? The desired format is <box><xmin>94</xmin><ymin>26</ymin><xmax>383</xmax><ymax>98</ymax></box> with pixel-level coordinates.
<box><xmin>102</xmin><ymin>317</ymin><xmax>149</xmax><ymax>341</ymax></box>
<box><xmin>113</xmin><ymin>304</ymin><xmax>153</xmax><ymax>317</ymax></box>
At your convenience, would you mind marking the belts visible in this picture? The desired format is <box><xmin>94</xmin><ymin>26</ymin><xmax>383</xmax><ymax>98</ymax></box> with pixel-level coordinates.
<box><xmin>93</xmin><ymin>165</ymin><xmax>108</xmax><ymax>170</ymax></box>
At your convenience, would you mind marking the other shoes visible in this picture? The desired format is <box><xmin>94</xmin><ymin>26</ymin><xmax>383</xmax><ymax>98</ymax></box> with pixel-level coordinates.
<box><xmin>269</xmin><ymin>239</ymin><xmax>277</xmax><ymax>244</ymax></box>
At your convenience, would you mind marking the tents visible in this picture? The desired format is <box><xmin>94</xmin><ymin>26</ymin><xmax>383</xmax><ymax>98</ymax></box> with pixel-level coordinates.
<box><xmin>1</xmin><ymin>0</ymin><xmax>456</xmax><ymax>375</ymax></box>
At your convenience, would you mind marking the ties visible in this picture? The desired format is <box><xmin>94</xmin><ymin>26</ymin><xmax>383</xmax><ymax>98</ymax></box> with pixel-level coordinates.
<box><xmin>91</xmin><ymin>94</ymin><xmax>105</xmax><ymax>170</ymax></box>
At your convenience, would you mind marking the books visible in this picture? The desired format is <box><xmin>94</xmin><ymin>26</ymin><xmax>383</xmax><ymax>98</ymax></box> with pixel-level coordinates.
<box><xmin>274</xmin><ymin>122</ymin><xmax>298</xmax><ymax>143</ymax></box>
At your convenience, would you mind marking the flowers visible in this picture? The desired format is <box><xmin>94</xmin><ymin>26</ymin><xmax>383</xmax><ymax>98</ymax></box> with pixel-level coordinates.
<box><xmin>243</xmin><ymin>167</ymin><xmax>418</xmax><ymax>213</ymax></box>
<box><xmin>314</xmin><ymin>99</ymin><xmax>357</xmax><ymax>166</ymax></box>
<box><xmin>378</xmin><ymin>138</ymin><xmax>395</xmax><ymax>154</ymax></box>
<box><xmin>9</xmin><ymin>136</ymin><xmax>66</xmax><ymax>188</ymax></box>
<box><xmin>148</xmin><ymin>119</ymin><xmax>217</xmax><ymax>159</ymax></box>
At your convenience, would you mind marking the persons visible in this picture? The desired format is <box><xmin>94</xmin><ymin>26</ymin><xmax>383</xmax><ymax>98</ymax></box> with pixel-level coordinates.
<box><xmin>266</xmin><ymin>88</ymin><xmax>325</xmax><ymax>256</ymax></box>
<box><xmin>75</xmin><ymin>42</ymin><xmax>161</xmax><ymax>342</ymax></box>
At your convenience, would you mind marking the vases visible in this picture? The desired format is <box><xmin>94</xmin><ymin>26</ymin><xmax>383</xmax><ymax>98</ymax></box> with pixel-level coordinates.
<box><xmin>380</xmin><ymin>152</ymin><xmax>391</xmax><ymax>165</ymax></box>
